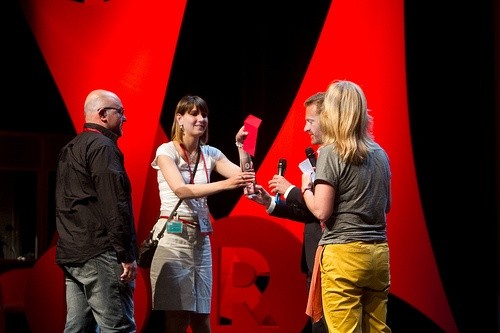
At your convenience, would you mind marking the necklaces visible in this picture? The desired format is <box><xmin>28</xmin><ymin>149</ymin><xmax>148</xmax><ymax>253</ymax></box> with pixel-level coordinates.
<box><xmin>187</xmin><ymin>152</ymin><xmax>197</xmax><ymax>163</ymax></box>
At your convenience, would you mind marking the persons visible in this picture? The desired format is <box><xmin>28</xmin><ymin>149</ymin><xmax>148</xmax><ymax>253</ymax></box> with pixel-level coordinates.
<box><xmin>56</xmin><ymin>89</ymin><xmax>139</xmax><ymax>333</ymax></box>
<box><xmin>151</xmin><ymin>96</ymin><xmax>256</xmax><ymax>333</ymax></box>
<box><xmin>244</xmin><ymin>94</ymin><xmax>330</xmax><ymax>333</ymax></box>
<box><xmin>302</xmin><ymin>80</ymin><xmax>390</xmax><ymax>333</ymax></box>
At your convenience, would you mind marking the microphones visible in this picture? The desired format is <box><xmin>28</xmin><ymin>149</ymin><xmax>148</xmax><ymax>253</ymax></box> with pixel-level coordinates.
<box><xmin>275</xmin><ymin>159</ymin><xmax>286</xmax><ymax>202</ymax></box>
<box><xmin>306</xmin><ymin>148</ymin><xmax>317</xmax><ymax>172</ymax></box>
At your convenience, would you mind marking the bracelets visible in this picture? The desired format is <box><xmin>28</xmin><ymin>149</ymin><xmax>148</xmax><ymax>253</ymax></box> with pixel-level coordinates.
<box><xmin>302</xmin><ymin>187</ymin><xmax>313</xmax><ymax>195</ymax></box>
<box><xmin>235</xmin><ymin>142</ymin><xmax>244</xmax><ymax>147</ymax></box>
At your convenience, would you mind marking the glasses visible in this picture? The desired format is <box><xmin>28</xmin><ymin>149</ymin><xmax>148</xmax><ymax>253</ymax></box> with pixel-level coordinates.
<box><xmin>97</xmin><ymin>107</ymin><xmax>123</xmax><ymax>116</ymax></box>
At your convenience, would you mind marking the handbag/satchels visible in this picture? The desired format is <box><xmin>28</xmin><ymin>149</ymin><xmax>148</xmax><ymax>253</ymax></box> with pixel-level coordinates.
<box><xmin>136</xmin><ymin>236</ymin><xmax>158</xmax><ymax>269</ymax></box>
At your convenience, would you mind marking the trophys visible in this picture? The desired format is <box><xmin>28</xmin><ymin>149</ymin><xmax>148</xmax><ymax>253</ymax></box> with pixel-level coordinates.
<box><xmin>241</xmin><ymin>158</ymin><xmax>257</xmax><ymax>196</ymax></box>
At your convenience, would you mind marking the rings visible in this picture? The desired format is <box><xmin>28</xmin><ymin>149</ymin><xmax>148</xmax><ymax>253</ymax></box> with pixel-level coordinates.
<box><xmin>241</xmin><ymin>176</ymin><xmax>244</xmax><ymax>180</ymax></box>
